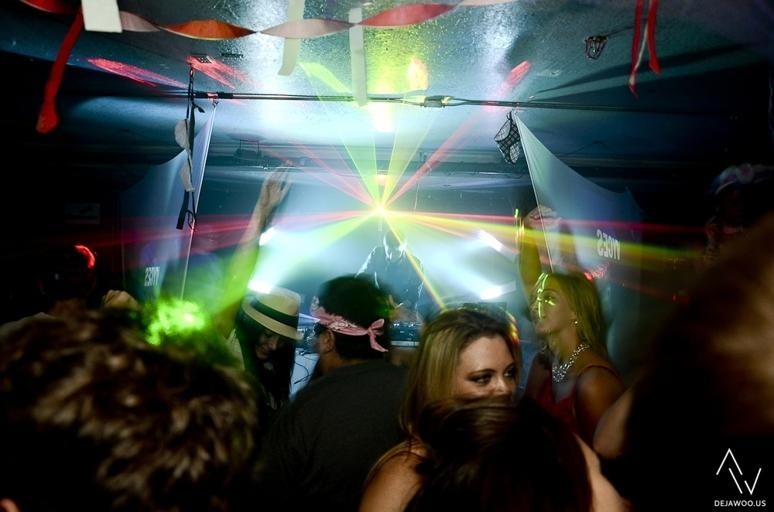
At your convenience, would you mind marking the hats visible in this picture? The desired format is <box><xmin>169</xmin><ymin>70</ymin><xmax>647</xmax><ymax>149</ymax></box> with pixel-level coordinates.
<box><xmin>238</xmin><ymin>286</ymin><xmax>307</xmax><ymax>340</ymax></box>
